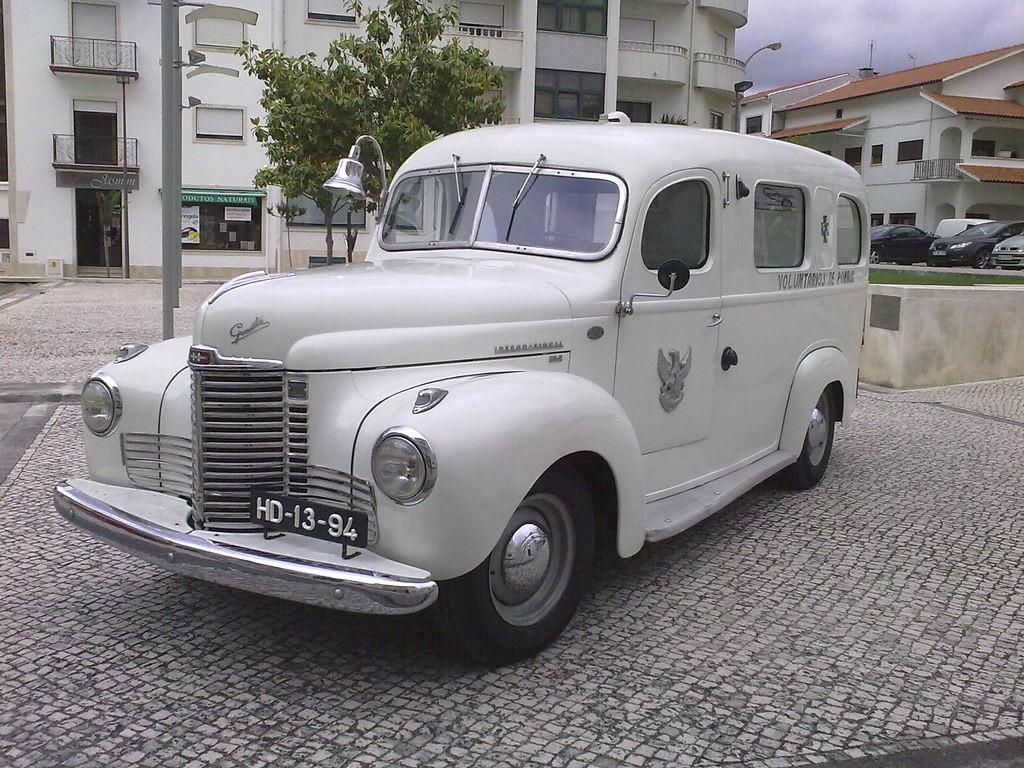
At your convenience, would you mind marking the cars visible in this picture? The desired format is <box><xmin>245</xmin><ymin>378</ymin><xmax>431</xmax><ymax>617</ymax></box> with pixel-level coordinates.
<box><xmin>48</xmin><ymin>118</ymin><xmax>873</xmax><ymax>663</ymax></box>
<box><xmin>989</xmin><ymin>230</ymin><xmax>1024</xmax><ymax>271</ymax></box>
<box><xmin>869</xmin><ymin>223</ymin><xmax>943</xmax><ymax>266</ymax></box>
<box><xmin>926</xmin><ymin>220</ymin><xmax>1024</xmax><ymax>268</ymax></box>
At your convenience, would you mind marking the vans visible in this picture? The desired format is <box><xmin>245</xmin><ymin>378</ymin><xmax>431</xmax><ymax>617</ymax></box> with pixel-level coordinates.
<box><xmin>933</xmin><ymin>218</ymin><xmax>998</xmax><ymax>236</ymax></box>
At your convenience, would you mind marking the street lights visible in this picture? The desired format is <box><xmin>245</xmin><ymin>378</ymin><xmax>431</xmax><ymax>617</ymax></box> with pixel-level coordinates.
<box><xmin>733</xmin><ymin>80</ymin><xmax>754</xmax><ymax>133</ymax></box>
